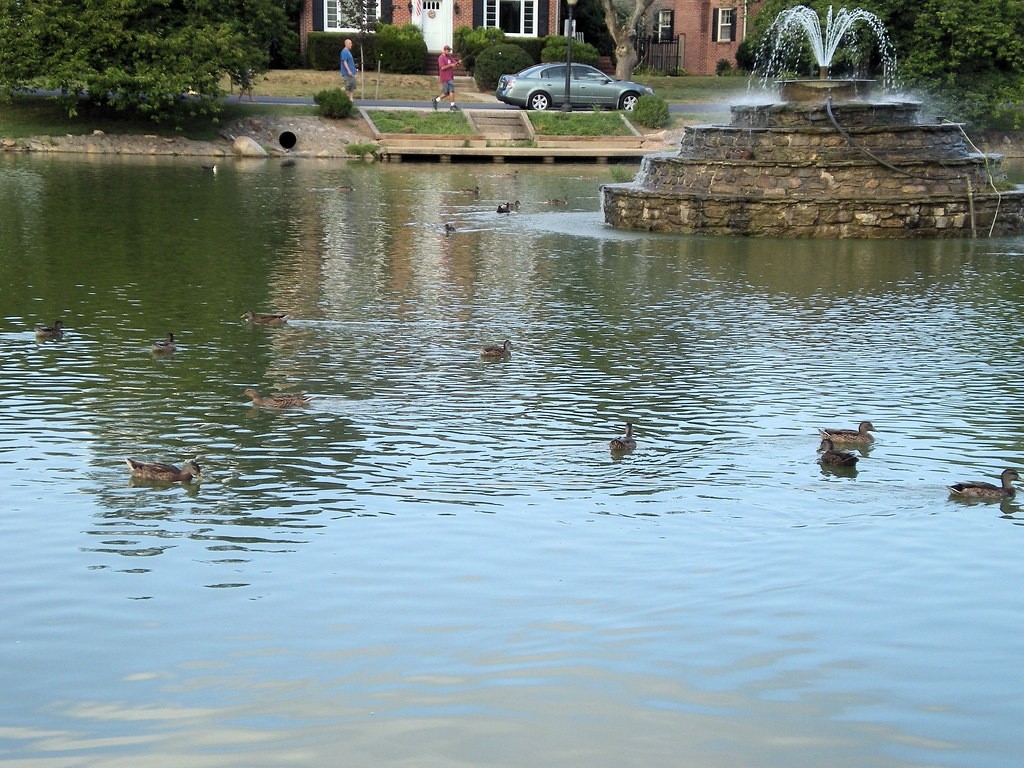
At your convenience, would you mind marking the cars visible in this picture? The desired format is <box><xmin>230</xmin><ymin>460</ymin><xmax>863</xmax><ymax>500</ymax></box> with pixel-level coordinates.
<box><xmin>496</xmin><ymin>62</ymin><xmax>656</xmax><ymax>113</ymax></box>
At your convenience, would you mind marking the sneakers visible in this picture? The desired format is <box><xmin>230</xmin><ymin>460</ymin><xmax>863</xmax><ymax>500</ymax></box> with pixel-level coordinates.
<box><xmin>451</xmin><ymin>105</ymin><xmax>458</xmax><ymax>111</ymax></box>
<box><xmin>432</xmin><ymin>98</ymin><xmax>439</xmax><ymax>110</ymax></box>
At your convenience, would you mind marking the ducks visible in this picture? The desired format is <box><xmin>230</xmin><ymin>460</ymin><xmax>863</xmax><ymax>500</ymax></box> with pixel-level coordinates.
<box><xmin>240</xmin><ymin>309</ymin><xmax>290</xmax><ymax>325</ymax></box>
<box><xmin>609</xmin><ymin>422</ymin><xmax>636</xmax><ymax>451</ymax></box>
<box><xmin>818</xmin><ymin>421</ymin><xmax>879</xmax><ymax>443</ymax></box>
<box><xmin>479</xmin><ymin>340</ymin><xmax>513</xmax><ymax>356</ymax></box>
<box><xmin>945</xmin><ymin>469</ymin><xmax>1024</xmax><ymax>498</ymax></box>
<box><xmin>496</xmin><ymin>200</ymin><xmax>522</xmax><ymax>213</ymax></box>
<box><xmin>237</xmin><ymin>388</ymin><xmax>316</xmax><ymax>409</ymax></box>
<box><xmin>816</xmin><ymin>439</ymin><xmax>859</xmax><ymax>465</ymax></box>
<box><xmin>34</xmin><ymin>320</ymin><xmax>66</xmax><ymax>340</ymax></box>
<box><xmin>547</xmin><ymin>192</ymin><xmax>573</xmax><ymax>206</ymax></box>
<box><xmin>152</xmin><ymin>333</ymin><xmax>176</xmax><ymax>352</ymax></box>
<box><xmin>202</xmin><ymin>163</ymin><xmax>220</xmax><ymax>174</ymax></box>
<box><xmin>125</xmin><ymin>457</ymin><xmax>204</xmax><ymax>482</ymax></box>
<box><xmin>334</xmin><ymin>183</ymin><xmax>358</xmax><ymax>193</ymax></box>
<box><xmin>458</xmin><ymin>186</ymin><xmax>481</xmax><ymax>197</ymax></box>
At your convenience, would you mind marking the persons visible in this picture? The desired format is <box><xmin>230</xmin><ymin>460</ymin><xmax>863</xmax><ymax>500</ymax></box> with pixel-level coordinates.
<box><xmin>340</xmin><ymin>39</ymin><xmax>358</xmax><ymax>103</ymax></box>
<box><xmin>432</xmin><ymin>45</ymin><xmax>462</xmax><ymax>112</ymax></box>
<box><xmin>236</xmin><ymin>67</ymin><xmax>256</xmax><ymax>103</ymax></box>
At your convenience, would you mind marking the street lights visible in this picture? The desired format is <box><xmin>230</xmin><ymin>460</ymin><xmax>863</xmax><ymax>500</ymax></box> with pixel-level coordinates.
<box><xmin>561</xmin><ymin>0</ymin><xmax>579</xmax><ymax>113</ymax></box>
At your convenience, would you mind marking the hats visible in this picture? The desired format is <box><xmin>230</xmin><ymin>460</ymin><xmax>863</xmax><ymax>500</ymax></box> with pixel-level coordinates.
<box><xmin>443</xmin><ymin>46</ymin><xmax>453</xmax><ymax>51</ymax></box>
